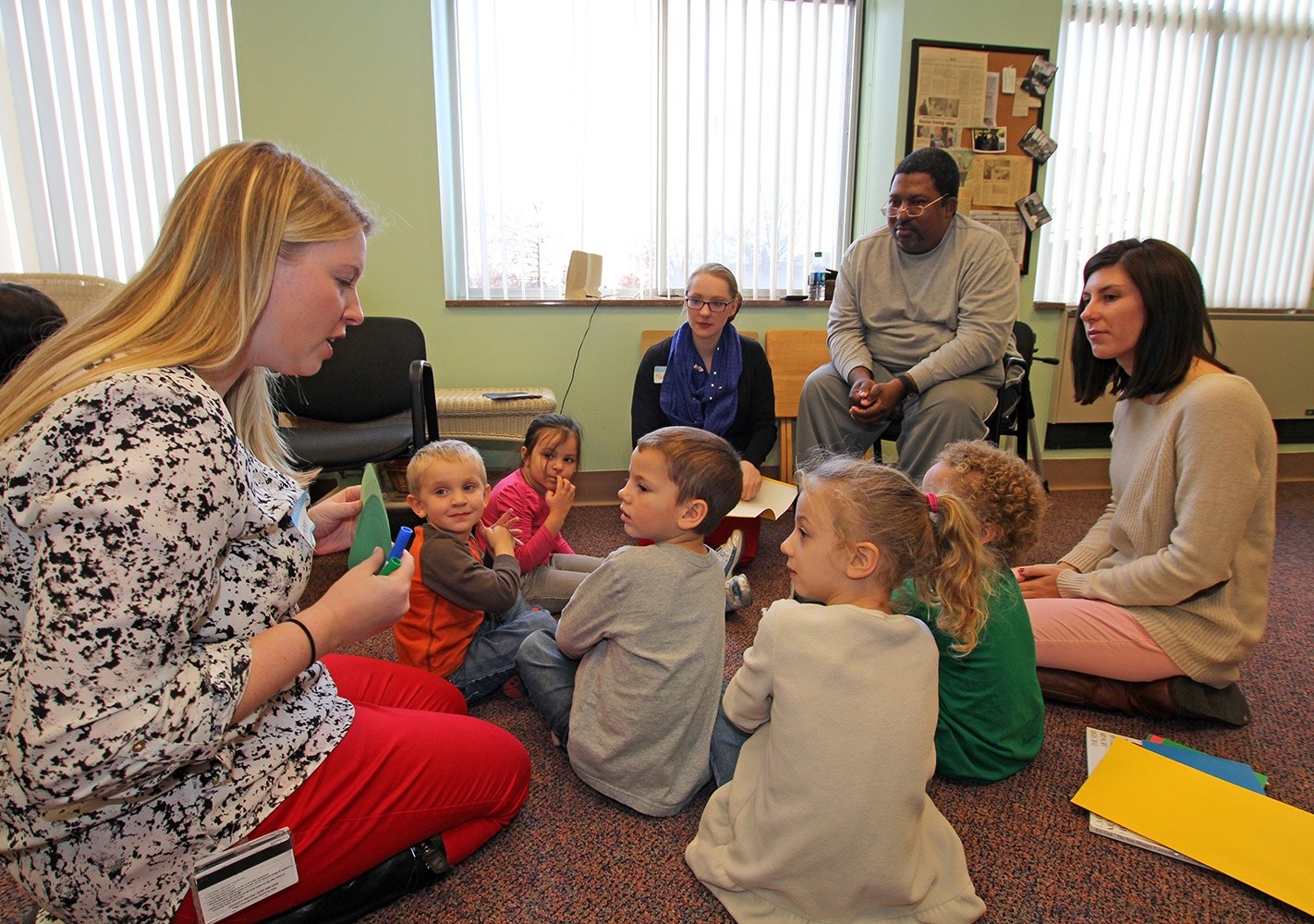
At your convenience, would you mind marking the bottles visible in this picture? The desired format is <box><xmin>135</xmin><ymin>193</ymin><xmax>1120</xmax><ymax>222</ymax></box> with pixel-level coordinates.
<box><xmin>807</xmin><ymin>252</ymin><xmax>825</xmax><ymax>302</ymax></box>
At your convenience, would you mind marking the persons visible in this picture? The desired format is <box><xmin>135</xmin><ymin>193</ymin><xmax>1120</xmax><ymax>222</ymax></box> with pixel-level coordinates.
<box><xmin>627</xmin><ymin>260</ymin><xmax>780</xmax><ymax>569</ymax></box>
<box><xmin>790</xmin><ymin>145</ymin><xmax>1022</xmax><ymax>496</ymax></box>
<box><xmin>887</xmin><ymin>440</ymin><xmax>1054</xmax><ymax>787</ymax></box>
<box><xmin>512</xmin><ymin>426</ymin><xmax>747</xmax><ymax>817</ymax></box>
<box><xmin>2</xmin><ymin>135</ymin><xmax>534</xmax><ymax>924</ymax></box>
<box><xmin>682</xmin><ymin>443</ymin><xmax>1019</xmax><ymax>924</ymax></box>
<box><xmin>474</xmin><ymin>412</ymin><xmax>755</xmax><ymax>621</ymax></box>
<box><xmin>388</xmin><ymin>439</ymin><xmax>559</xmax><ymax>709</ymax></box>
<box><xmin>997</xmin><ymin>238</ymin><xmax>1281</xmax><ymax>728</ymax></box>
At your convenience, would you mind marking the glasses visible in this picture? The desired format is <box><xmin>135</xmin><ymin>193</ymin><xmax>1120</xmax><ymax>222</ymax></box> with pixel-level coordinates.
<box><xmin>684</xmin><ymin>290</ymin><xmax>735</xmax><ymax>313</ymax></box>
<box><xmin>880</xmin><ymin>194</ymin><xmax>950</xmax><ymax>218</ymax></box>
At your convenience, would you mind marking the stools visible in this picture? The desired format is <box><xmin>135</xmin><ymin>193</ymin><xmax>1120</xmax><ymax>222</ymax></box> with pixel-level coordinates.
<box><xmin>429</xmin><ymin>385</ymin><xmax>556</xmax><ymax>442</ymax></box>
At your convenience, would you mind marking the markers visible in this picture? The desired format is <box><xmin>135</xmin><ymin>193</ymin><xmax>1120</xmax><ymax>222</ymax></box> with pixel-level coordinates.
<box><xmin>381</xmin><ymin>527</ymin><xmax>413</xmax><ymax>575</ymax></box>
<box><xmin>377</xmin><ymin>557</ymin><xmax>403</xmax><ymax>577</ymax></box>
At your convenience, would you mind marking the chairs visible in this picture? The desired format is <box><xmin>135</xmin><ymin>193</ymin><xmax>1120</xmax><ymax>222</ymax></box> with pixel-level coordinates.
<box><xmin>874</xmin><ymin>312</ymin><xmax>1048</xmax><ymax>487</ymax></box>
<box><xmin>264</xmin><ymin>311</ymin><xmax>440</xmax><ymax>472</ymax></box>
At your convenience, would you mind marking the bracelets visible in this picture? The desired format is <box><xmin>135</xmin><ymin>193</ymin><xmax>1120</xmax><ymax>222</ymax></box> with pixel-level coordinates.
<box><xmin>284</xmin><ymin>617</ymin><xmax>317</xmax><ymax>668</ymax></box>
<box><xmin>896</xmin><ymin>374</ymin><xmax>914</xmax><ymax>400</ymax></box>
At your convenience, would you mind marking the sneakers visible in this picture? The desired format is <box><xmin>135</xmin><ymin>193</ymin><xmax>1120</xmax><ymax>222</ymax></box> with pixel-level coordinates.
<box><xmin>716</xmin><ymin>529</ymin><xmax>742</xmax><ymax>582</ymax></box>
<box><xmin>724</xmin><ymin>574</ymin><xmax>752</xmax><ymax>613</ymax></box>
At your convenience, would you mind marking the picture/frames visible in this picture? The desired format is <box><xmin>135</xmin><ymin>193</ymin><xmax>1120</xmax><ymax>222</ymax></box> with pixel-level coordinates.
<box><xmin>902</xmin><ymin>35</ymin><xmax>1055</xmax><ymax>276</ymax></box>
